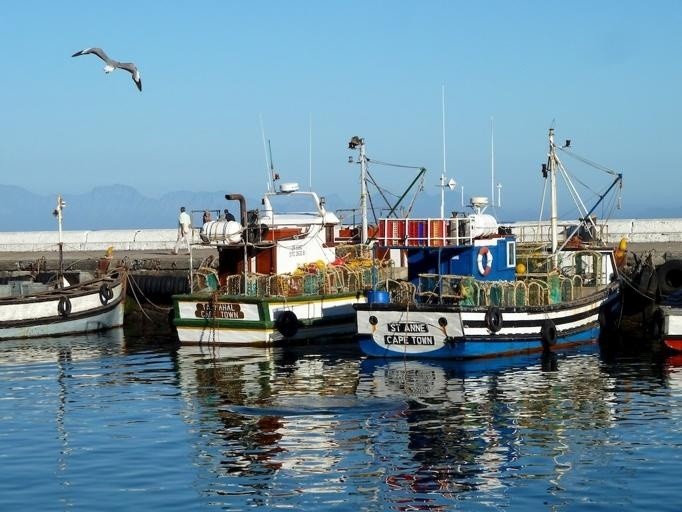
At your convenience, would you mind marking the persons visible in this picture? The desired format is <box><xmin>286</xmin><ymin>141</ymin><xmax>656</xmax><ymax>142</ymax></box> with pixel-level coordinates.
<box><xmin>171</xmin><ymin>206</ymin><xmax>191</xmax><ymax>256</ymax></box>
<box><xmin>224</xmin><ymin>208</ymin><xmax>235</xmax><ymax>222</ymax></box>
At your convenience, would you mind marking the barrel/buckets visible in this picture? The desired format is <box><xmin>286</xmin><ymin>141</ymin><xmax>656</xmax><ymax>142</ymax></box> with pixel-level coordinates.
<box><xmin>368</xmin><ymin>291</ymin><xmax>391</xmax><ymax>304</ymax></box>
<box><xmin>201</xmin><ymin>221</ymin><xmax>244</xmax><ymax>245</ymax></box>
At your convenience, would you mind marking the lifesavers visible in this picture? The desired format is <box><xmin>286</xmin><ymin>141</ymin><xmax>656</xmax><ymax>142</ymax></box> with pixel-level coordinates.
<box><xmin>478</xmin><ymin>248</ymin><xmax>492</xmax><ymax>275</ymax></box>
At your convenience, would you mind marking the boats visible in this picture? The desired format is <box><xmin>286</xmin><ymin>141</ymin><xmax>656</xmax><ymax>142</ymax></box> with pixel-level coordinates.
<box><xmin>350</xmin><ymin>118</ymin><xmax>650</xmax><ymax>362</ymax></box>
<box><xmin>0</xmin><ymin>301</ymin><xmax>124</xmax><ymax>339</ymax></box>
<box><xmin>171</xmin><ymin>86</ymin><xmax>519</xmax><ymax>347</ymax></box>
<box><xmin>0</xmin><ymin>271</ymin><xmax>122</xmax><ymax>321</ymax></box>
<box><xmin>637</xmin><ymin>247</ymin><xmax>682</xmax><ymax>352</ymax></box>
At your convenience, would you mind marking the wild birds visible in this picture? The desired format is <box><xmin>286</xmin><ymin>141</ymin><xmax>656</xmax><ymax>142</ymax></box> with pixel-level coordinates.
<box><xmin>71</xmin><ymin>47</ymin><xmax>141</xmax><ymax>92</ymax></box>
